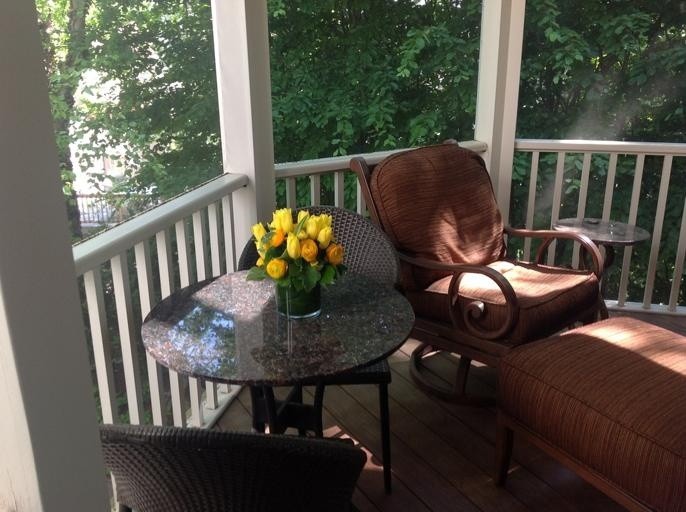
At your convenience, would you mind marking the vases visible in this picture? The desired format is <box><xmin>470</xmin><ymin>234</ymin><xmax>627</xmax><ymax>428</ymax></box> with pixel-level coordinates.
<box><xmin>275</xmin><ymin>282</ymin><xmax>322</xmax><ymax>319</ymax></box>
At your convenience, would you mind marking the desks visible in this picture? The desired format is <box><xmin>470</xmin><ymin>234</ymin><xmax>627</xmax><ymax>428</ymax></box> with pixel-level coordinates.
<box><xmin>141</xmin><ymin>269</ymin><xmax>415</xmax><ymax>437</ymax></box>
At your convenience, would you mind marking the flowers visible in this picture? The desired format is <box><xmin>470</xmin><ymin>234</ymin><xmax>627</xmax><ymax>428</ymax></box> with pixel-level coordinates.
<box><xmin>246</xmin><ymin>208</ymin><xmax>347</xmax><ymax>293</ymax></box>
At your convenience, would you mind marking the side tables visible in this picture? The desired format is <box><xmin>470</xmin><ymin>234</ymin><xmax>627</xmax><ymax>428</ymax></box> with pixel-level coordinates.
<box><xmin>553</xmin><ymin>217</ymin><xmax>651</xmax><ymax>321</ymax></box>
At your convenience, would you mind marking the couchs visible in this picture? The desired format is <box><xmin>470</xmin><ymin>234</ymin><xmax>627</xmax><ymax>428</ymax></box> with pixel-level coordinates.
<box><xmin>497</xmin><ymin>316</ymin><xmax>686</xmax><ymax>512</ymax></box>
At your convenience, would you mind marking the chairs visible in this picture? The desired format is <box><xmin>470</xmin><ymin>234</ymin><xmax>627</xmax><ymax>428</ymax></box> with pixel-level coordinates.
<box><xmin>99</xmin><ymin>424</ymin><xmax>367</xmax><ymax>512</ymax></box>
<box><xmin>350</xmin><ymin>138</ymin><xmax>603</xmax><ymax>405</ymax></box>
<box><xmin>238</xmin><ymin>205</ymin><xmax>402</xmax><ymax>493</ymax></box>
